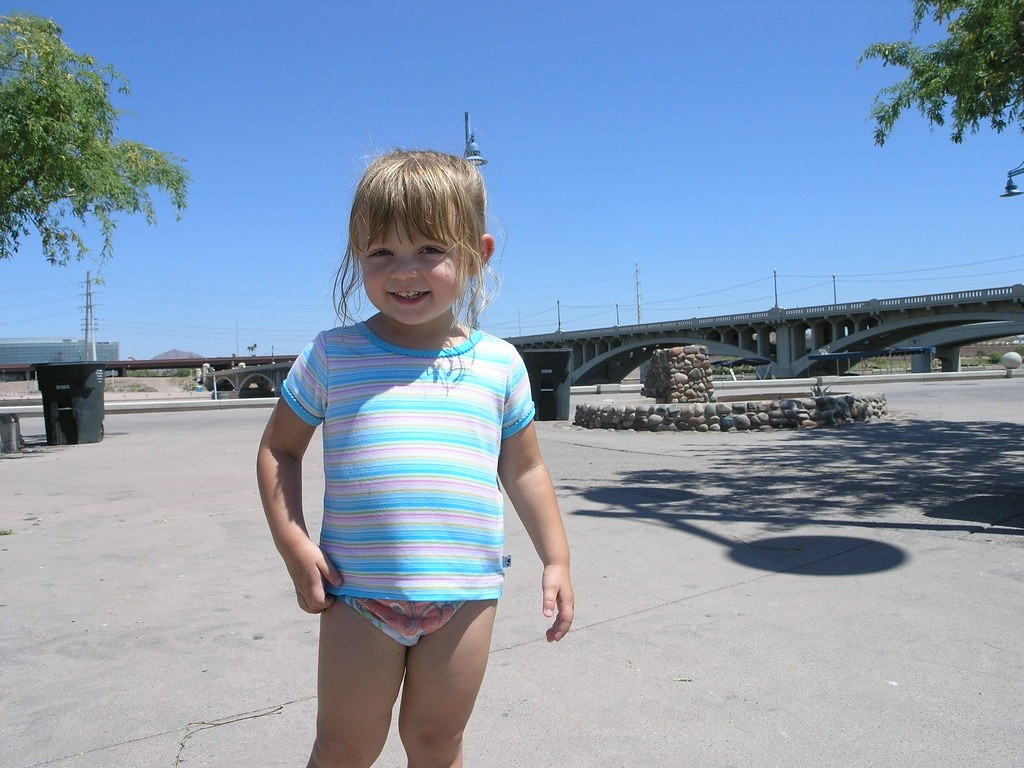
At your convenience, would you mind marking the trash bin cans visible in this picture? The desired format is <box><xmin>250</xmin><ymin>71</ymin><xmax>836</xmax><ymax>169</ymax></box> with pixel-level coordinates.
<box><xmin>0</xmin><ymin>413</ymin><xmax>21</xmax><ymax>452</ymax></box>
<box><xmin>522</xmin><ymin>348</ymin><xmax>573</xmax><ymax>421</ymax></box>
<box><xmin>31</xmin><ymin>361</ymin><xmax>107</xmax><ymax>446</ymax></box>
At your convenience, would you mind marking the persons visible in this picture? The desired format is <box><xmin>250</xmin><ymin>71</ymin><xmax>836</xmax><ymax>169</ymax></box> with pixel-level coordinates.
<box><xmin>256</xmin><ymin>149</ymin><xmax>574</xmax><ymax>768</ymax></box>
